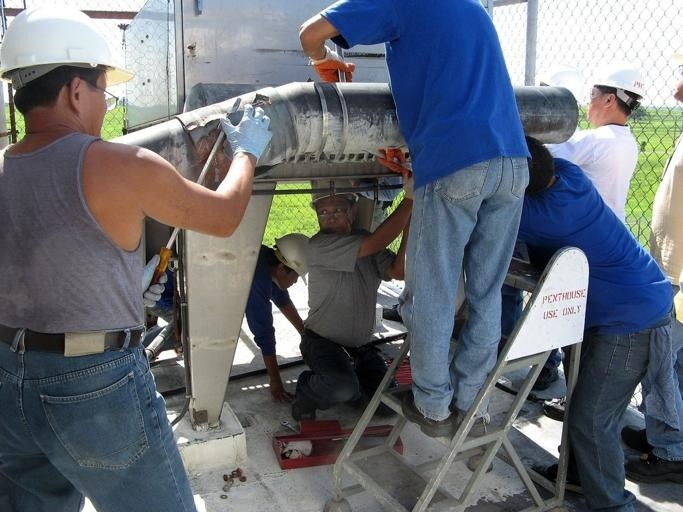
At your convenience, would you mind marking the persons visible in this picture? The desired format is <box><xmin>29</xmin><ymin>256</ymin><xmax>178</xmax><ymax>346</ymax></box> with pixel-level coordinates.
<box><xmin>299</xmin><ymin>0</ymin><xmax>530</xmax><ymax>437</ymax></box>
<box><xmin>293</xmin><ymin>173</ymin><xmax>417</xmax><ymax>422</ymax></box>
<box><xmin>531</xmin><ymin>75</ymin><xmax>645</xmax><ymax>421</ymax></box>
<box><xmin>145</xmin><ymin>233</ymin><xmax>310</xmax><ymax>403</ymax></box>
<box><xmin>516</xmin><ymin>136</ymin><xmax>679</xmax><ymax>512</ymax></box>
<box><xmin>622</xmin><ymin>65</ymin><xmax>683</xmax><ymax>483</ymax></box>
<box><xmin>0</xmin><ymin>3</ymin><xmax>273</xmax><ymax>512</ymax></box>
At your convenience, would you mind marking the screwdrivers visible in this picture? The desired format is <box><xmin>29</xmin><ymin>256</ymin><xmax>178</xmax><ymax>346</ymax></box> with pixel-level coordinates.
<box><xmin>152</xmin><ymin>97</ymin><xmax>241</xmax><ymax>283</ymax></box>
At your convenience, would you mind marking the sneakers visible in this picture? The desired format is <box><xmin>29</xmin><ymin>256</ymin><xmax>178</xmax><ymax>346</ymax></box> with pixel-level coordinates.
<box><xmin>532</xmin><ymin>460</ymin><xmax>584</xmax><ymax>495</ymax></box>
<box><xmin>292</xmin><ymin>370</ymin><xmax>316</xmax><ymax>422</ymax></box>
<box><xmin>621</xmin><ymin>425</ymin><xmax>650</xmax><ymax>449</ymax></box>
<box><xmin>454</xmin><ymin>407</ymin><xmax>486</xmax><ymax>437</ymax></box>
<box><xmin>532</xmin><ymin>367</ymin><xmax>560</xmax><ymax>390</ymax></box>
<box><xmin>401</xmin><ymin>389</ymin><xmax>454</xmax><ymax>437</ymax></box>
<box><xmin>624</xmin><ymin>450</ymin><xmax>683</xmax><ymax>486</ymax></box>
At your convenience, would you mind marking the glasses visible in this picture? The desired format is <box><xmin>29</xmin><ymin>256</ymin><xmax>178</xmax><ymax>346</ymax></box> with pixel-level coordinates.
<box><xmin>318</xmin><ymin>201</ymin><xmax>353</xmax><ymax>220</ymax></box>
<box><xmin>66</xmin><ymin>74</ymin><xmax>119</xmax><ymax>111</ymax></box>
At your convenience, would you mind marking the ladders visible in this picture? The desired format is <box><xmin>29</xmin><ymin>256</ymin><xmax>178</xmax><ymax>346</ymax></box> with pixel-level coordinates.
<box><xmin>333</xmin><ymin>246</ymin><xmax>589</xmax><ymax>512</ymax></box>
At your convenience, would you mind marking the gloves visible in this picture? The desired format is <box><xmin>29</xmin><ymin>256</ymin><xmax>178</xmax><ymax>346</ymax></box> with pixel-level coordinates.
<box><xmin>309</xmin><ymin>46</ymin><xmax>355</xmax><ymax>82</ymax></box>
<box><xmin>378</xmin><ymin>148</ymin><xmax>410</xmax><ymax>174</ymax></box>
<box><xmin>142</xmin><ymin>253</ymin><xmax>167</xmax><ymax>309</ymax></box>
<box><xmin>220</xmin><ymin>103</ymin><xmax>274</xmax><ymax>165</ymax></box>
<box><xmin>403</xmin><ymin>170</ymin><xmax>414</xmax><ymax>200</ymax></box>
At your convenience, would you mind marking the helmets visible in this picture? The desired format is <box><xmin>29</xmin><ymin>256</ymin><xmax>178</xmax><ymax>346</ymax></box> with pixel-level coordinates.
<box><xmin>540</xmin><ymin>65</ymin><xmax>585</xmax><ymax>106</ymax></box>
<box><xmin>0</xmin><ymin>5</ymin><xmax>135</xmax><ymax>88</ymax></box>
<box><xmin>672</xmin><ymin>51</ymin><xmax>683</xmax><ymax>64</ymax></box>
<box><xmin>310</xmin><ymin>179</ymin><xmax>359</xmax><ymax>210</ymax></box>
<box><xmin>592</xmin><ymin>61</ymin><xmax>646</xmax><ymax>100</ymax></box>
<box><xmin>275</xmin><ymin>233</ymin><xmax>310</xmax><ymax>285</ymax></box>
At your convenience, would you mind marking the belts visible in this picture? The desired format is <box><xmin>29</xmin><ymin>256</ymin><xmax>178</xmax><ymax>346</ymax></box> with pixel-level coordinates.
<box><xmin>0</xmin><ymin>324</ymin><xmax>144</xmax><ymax>354</ymax></box>
<box><xmin>649</xmin><ymin>303</ymin><xmax>675</xmax><ymax>329</ymax></box>
